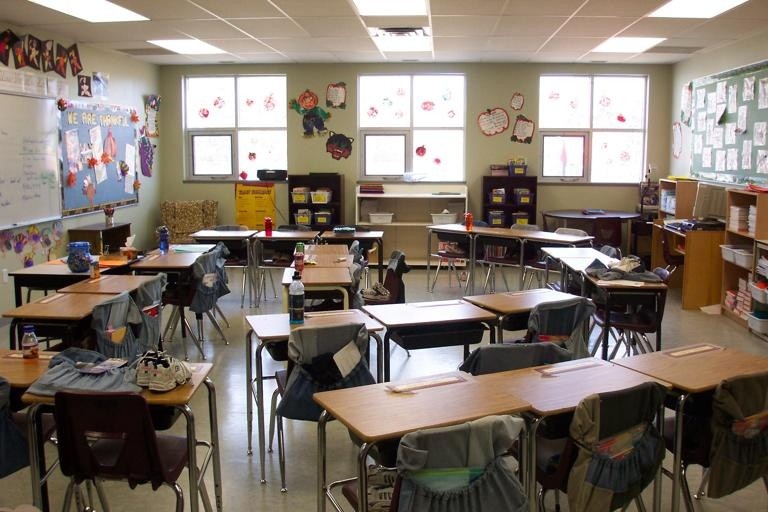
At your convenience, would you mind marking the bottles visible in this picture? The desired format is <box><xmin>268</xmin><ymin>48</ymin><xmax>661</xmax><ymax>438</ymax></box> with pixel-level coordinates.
<box><xmin>22</xmin><ymin>325</ymin><xmax>40</xmax><ymax>357</ymax></box>
<box><xmin>288</xmin><ymin>271</ymin><xmax>305</xmax><ymax>323</ymax></box>
<box><xmin>159</xmin><ymin>226</ymin><xmax>169</xmax><ymax>255</ymax></box>
<box><xmin>264</xmin><ymin>216</ymin><xmax>273</xmax><ymax>237</ymax></box>
<box><xmin>465</xmin><ymin>210</ymin><xmax>474</xmax><ymax>231</ymax></box>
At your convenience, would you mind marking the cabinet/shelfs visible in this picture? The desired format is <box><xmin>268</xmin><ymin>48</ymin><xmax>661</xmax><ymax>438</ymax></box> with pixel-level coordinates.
<box><xmin>355</xmin><ymin>185</ymin><xmax>469</xmax><ymax>269</ymax></box>
<box><xmin>657</xmin><ymin>178</ymin><xmax>699</xmax><ymax>219</ymax></box>
<box><xmin>481</xmin><ymin>175</ymin><xmax>538</xmax><ymax>260</ymax></box>
<box><xmin>719</xmin><ymin>189</ymin><xmax>768</xmax><ymax>327</ymax></box>
<box><xmin>287</xmin><ymin>174</ymin><xmax>345</xmax><ymax>230</ymax></box>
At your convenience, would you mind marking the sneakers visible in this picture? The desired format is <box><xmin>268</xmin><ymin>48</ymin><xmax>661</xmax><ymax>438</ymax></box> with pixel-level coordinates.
<box><xmin>373</xmin><ymin>281</ymin><xmax>390</xmax><ymax>295</ymax></box>
<box><xmin>444</xmin><ymin>244</ymin><xmax>465</xmax><ymax>255</ymax></box>
<box><xmin>461</xmin><ymin>271</ymin><xmax>467</xmax><ymax>280</ymax></box>
<box><xmin>360</xmin><ymin>288</ymin><xmax>376</xmax><ymax>295</ymax></box>
<box><xmin>128</xmin><ymin>349</ymin><xmax>163</xmax><ymax>386</ymax></box>
<box><xmin>148</xmin><ymin>356</ymin><xmax>194</xmax><ymax>391</ymax></box>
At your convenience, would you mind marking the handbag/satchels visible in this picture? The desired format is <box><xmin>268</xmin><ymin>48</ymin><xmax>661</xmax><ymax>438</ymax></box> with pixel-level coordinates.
<box><xmin>274</xmin><ymin>322</ymin><xmax>376</xmax><ymax>421</ymax></box>
<box><xmin>523</xmin><ymin>296</ymin><xmax>597</xmax><ymax>360</ymax></box>
<box><xmin>91</xmin><ymin>291</ymin><xmax>143</xmax><ymax>358</ymax></box>
<box><xmin>397</xmin><ymin>415</ymin><xmax>531</xmax><ymax>512</ymax></box>
<box><xmin>136</xmin><ymin>272</ymin><xmax>169</xmax><ymax>353</ymax></box>
<box><xmin>24</xmin><ymin>347</ymin><xmax>143</xmax><ymax>396</ymax></box>
<box><xmin>707</xmin><ymin>372</ymin><xmax>768</xmax><ymax>498</ymax></box>
<box><xmin>567</xmin><ymin>382</ymin><xmax>665</xmax><ymax>512</ymax></box>
<box><xmin>189</xmin><ymin>241</ymin><xmax>232</xmax><ymax>313</ymax></box>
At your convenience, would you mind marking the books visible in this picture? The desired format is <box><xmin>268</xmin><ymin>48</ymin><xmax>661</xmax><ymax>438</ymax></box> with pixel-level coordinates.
<box><xmin>507</xmin><ymin>156</ymin><xmax>529</xmax><ymax>176</ymax></box>
<box><xmin>483</xmin><ymin>187</ymin><xmax>531</xmax><ymax>257</ymax></box>
<box><xmin>660</xmin><ymin>188</ymin><xmax>676</xmax><ymax>212</ymax></box>
<box><xmin>724</xmin><ymin>203</ymin><xmax>768</xmax><ymax>334</ymax></box>
<box><xmin>356</xmin><ymin>179</ymin><xmax>385</xmax><ymax>194</ymax></box>
<box><xmin>432</xmin><ymin>192</ymin><xmax>461</xmax><ymax>195</ymax></box>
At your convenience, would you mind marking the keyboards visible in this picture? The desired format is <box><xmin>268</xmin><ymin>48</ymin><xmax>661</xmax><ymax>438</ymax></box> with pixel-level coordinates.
<box><xmin>666</xmin><ymin>222</ymin><xmax>697</xmax><ymax>233</ymax></box>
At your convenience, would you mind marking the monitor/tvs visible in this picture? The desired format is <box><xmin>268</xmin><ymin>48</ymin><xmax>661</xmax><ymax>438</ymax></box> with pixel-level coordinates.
<box><xmin>693</xmin><ymin>182</ymin><xmax>727</xmax><ymax>228</ymax></box>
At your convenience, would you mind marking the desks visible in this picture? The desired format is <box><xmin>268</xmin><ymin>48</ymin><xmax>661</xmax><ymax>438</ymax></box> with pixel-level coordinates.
<box><xmin>540</xmin><ymin>209</ymin><xmax>641</xmax><ymax>256</ymax></box>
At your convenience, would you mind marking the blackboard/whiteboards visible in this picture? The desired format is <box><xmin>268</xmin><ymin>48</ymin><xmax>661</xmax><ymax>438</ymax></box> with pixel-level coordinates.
<box><xmin>1</xmin><ymin>90</ymin><xmax>64</xmax><ymax>230</ymax></box>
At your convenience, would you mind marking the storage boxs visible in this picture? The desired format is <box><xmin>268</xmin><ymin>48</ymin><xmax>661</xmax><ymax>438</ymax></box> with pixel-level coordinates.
<box><xmin>488</xmin><ymin>193</ymin><xmax>534</xmax><ymax>227</ymax></box>
<box><xmin>367</xmin><ymin>212</ymin><xmax>394</xmax><ymax>223</ymax></box>
<box><xmin>290</xmin><ymin>191</ymin><xmax>331</xmax><ymax>226</ymax></box>
<box><xmin>430</xmin><ymin>211</ymin><xmax>458</xmax><ymax>224</ymax></box>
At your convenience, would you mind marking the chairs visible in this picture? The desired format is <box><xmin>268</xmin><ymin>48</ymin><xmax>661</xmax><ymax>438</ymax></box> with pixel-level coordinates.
<box><xmin>154</xmin><ymin>199</ymin><xmax>218</xmax><ymax>244</ymax></box>
